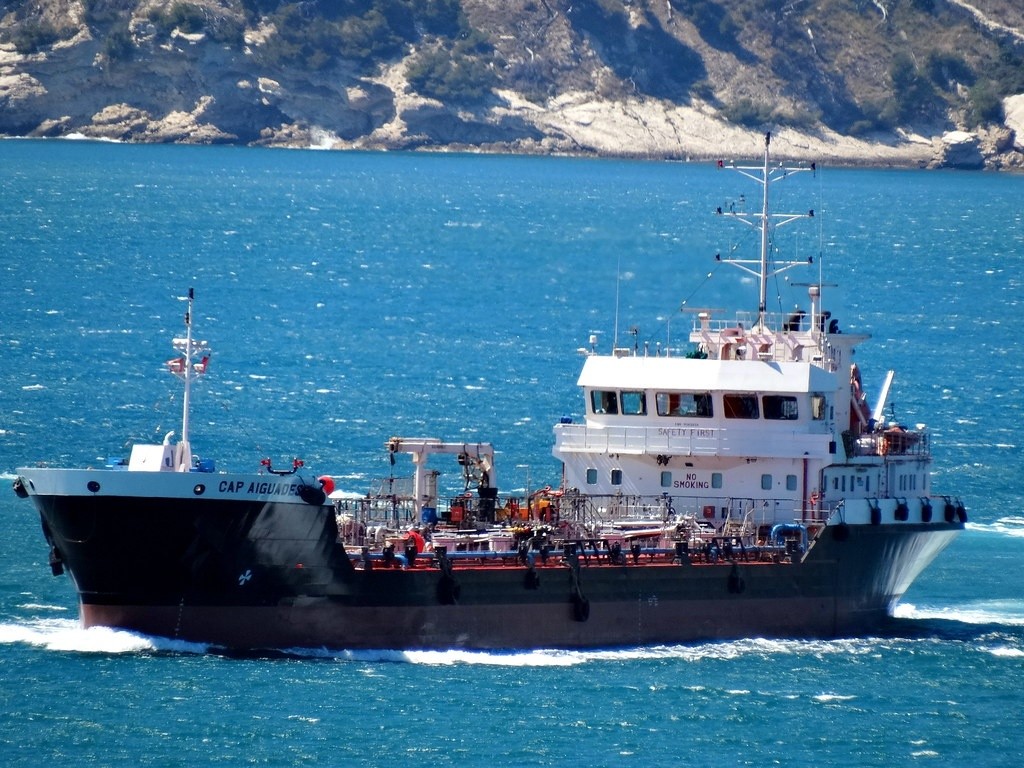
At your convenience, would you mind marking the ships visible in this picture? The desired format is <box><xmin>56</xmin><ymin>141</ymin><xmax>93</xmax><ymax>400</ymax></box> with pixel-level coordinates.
<box><xmin>12</xmin><ymin>124</ymin><xmax>972</xmax><ymax>655</ymax></box>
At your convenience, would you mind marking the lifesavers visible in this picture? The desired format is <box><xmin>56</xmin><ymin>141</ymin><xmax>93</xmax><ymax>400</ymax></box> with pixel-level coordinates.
<box><xmin>570</xmin><ymin>596</ymin><xmax>591</xmax><ymax>623</ymax></box>
